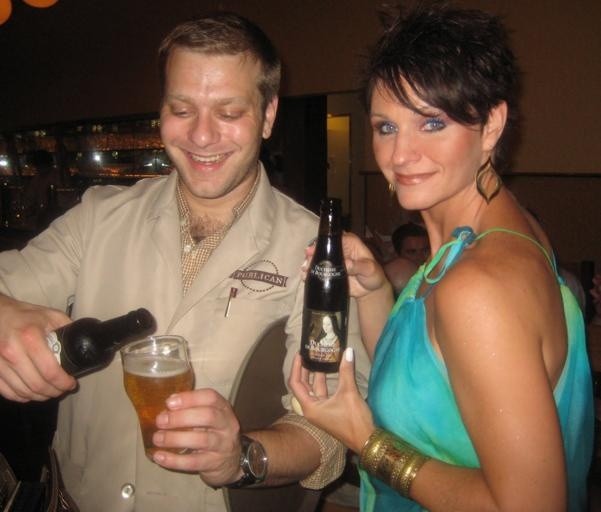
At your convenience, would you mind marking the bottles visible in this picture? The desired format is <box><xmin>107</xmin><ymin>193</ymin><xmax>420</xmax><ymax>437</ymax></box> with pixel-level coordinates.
<box><xmin>302</xmin><ymin>199</ymin><xmax>351</xmax><ymax>373</ymax></box>
<box><xmin>47</xmin><ymin>308</ymin><xmax>159</xmax><ymax>378</ymax></box>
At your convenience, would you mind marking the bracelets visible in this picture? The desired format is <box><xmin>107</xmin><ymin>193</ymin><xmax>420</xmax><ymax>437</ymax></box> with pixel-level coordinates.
<box><xmin>358</xmin><ymin>427</ymin><xmax>432</xmax><ymax>501</ymax></box>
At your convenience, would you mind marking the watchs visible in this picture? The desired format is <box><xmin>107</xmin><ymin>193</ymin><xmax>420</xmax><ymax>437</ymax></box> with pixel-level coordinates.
<box><xmin>223</xmin><ymin>434</ymin><xmax>268</xmax><ymax>490</ymax></box>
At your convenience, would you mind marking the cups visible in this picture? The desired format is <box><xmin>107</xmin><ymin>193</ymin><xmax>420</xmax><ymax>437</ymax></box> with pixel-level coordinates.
<box><xmin>120</xmin><ymin>336</ymin><xmax>195</xmax><ymax>463</ymax></box>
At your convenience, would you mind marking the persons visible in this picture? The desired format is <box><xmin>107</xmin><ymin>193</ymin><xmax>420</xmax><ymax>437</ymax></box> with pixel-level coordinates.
<box><xmin>383</xmin><ymin>258</ymin><xmax>419</xmax><ymax>295</ymax></box>
<box><xmin>309</xmin><ymin>314</ymin><xmax>342</xmax><ymax>362</ymax></box>
<box><xmin>1</xmin><ymin>11</ymin><xmax>372</xmax><ymax>512</ymax></box>
<box><xmin>288</xmin><ymin>8</ymin><xmax>593</xmax><ymax>512</ymax></box>
<box><xmin>392</xmin><ymin>224</ymin><xmax>429</xmax><ymax>268</ymax></box>
<box><xmin>589</xmin><ymin>274</ymin><xmax>601</xmax><ymax>374</ymax></box>
<box><xmin>27</xmin><ymin>149</ymin><xmax>67</xmax><ymax>238</ymax></box>
